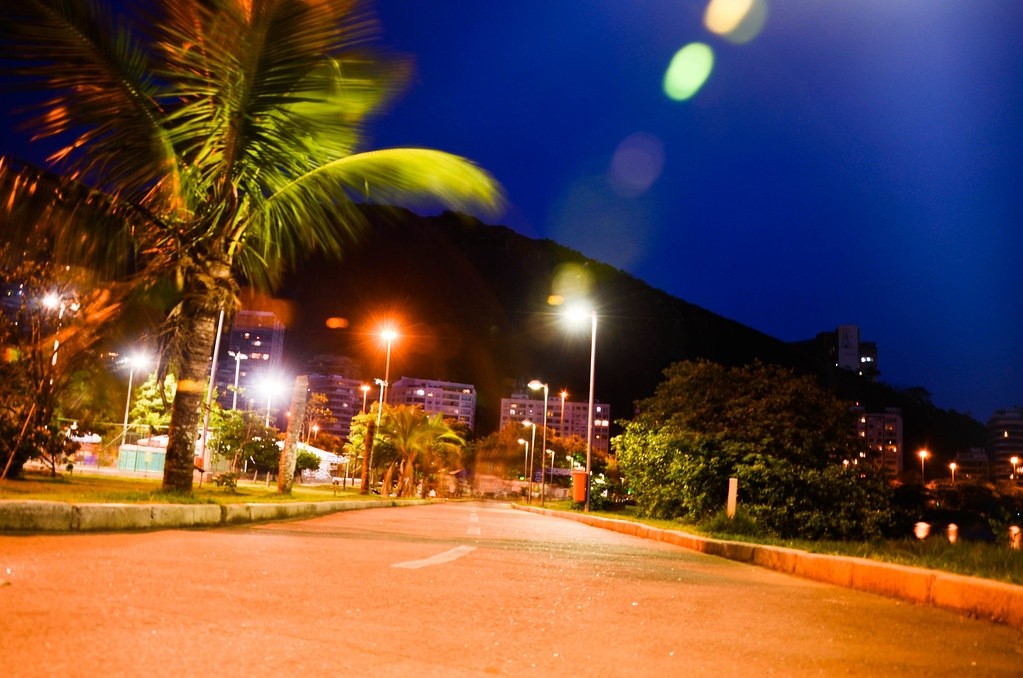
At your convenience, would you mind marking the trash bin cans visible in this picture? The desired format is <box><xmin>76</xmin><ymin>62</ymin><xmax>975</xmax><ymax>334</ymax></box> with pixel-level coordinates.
<box><xmin>571</xmin><ymin>471</ymin><xmax>588</xmax><ymax>504</ymax></box>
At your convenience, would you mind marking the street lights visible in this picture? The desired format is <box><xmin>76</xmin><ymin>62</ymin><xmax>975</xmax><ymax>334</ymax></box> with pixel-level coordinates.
<box><xmin>558</xmin><ymin>389</ymin><xmax>568</xmax><ymax>439</ymax></box>
<box><xmin>519</xmin><ymin>438</ymin><xmax>529</xmax><ymax>481</ymax></box>
<box><xmin>920</xmin><ymin>450</ymin><xmax>928</xmax><ymax>483</ymax></box>
<box><xmin>527</xmin><ymin>379</ymin><xmax>549</xmax><ymax>506</ymax></box>
<box><xmin>119</xmin><ymin>352</ymin><xmax>145</xmax><ymax>447</ymax></box>
<box><xmin>38</xmin><ymin>291</ymin><xmax>80</xmax><ymax>390</ymax></box>
<box><xmin>1010</xmin><ymin>456</ymin><xmax>1017</xmax><ymax>480</ymax></box>
<box><xmin>545</xmin><ymin>449</ymin><xmax>555</xmax><ymax>487</ymax></box>
<box><xmin>313</xmin><ymin>426</ymin><xmax>320</xmax><ymax>441</ymax></box>
<box><xmin>375</xmin><ymin>379</ymin><xmax>390</xmax><ymax>428</ymax></box>
<box><xmin>380</xmin><ymin>326</ymin><xmax>399</xmax><ymax>405</ymax></box>
<box><xmin>233</xmin><ymin>352</ymin><xmax>248</xmax><ymax>412</ymax></box>
<box><xmin>559</xmin><ymin>307</ymin><xmax>598</xmax><ymax>513</ymax></box>
<box><xmin>565</xmin><ymin>455</ymin><xmax>574</xmax><ymax>474</ymax></box>
<box><xmin>522</xmin><ymin>420</ymin><xmax>536</xmax><ymax>505</ymax></box>
<box><xmin>359</xmin><ymin>386</ymin><xmax>371</xmax><ymax>415</ymax></box>
<box><xmin>950</xmin><ymin>462</ymin><xmax>958</xmax><ymax>484</ymax></box>
